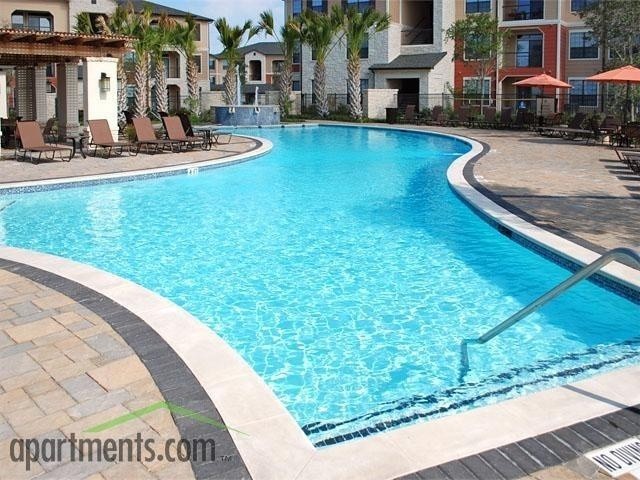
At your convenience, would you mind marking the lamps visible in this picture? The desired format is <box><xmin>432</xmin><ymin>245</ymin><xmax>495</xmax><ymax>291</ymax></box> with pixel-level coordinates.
<box><xmin>99</xmin><ymin>72</ymin><xmax>110</xmax><ymax>92</ymax></box>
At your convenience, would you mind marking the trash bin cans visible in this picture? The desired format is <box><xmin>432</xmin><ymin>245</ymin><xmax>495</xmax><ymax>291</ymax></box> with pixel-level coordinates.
<box><xmin>386</xmin><ymin>108</ymin><xmax>398</xmax><ymax>124</ymax></box>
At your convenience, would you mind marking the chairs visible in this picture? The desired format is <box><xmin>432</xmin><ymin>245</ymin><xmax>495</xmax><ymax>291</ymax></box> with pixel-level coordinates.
<box><xmin>397</xmin><ymin>105</ymin><xmax>640</xmax><ymax>175</ymax></box>
<box><xmin>0</xmin><ymin>111</ymin><xmax>234</xmax><ymax>165</ymax></box>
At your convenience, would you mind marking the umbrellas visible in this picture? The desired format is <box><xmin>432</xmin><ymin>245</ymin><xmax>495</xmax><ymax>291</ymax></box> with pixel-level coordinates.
<box><xmin>585</xmin><ymin>64</ymin><xmax>640</xmax><ymax>124</ymax></box>
<box><xmin>511</xmin><ymin>71</ymin><xmax>574</xmax><ymax>134</ymax></box>
